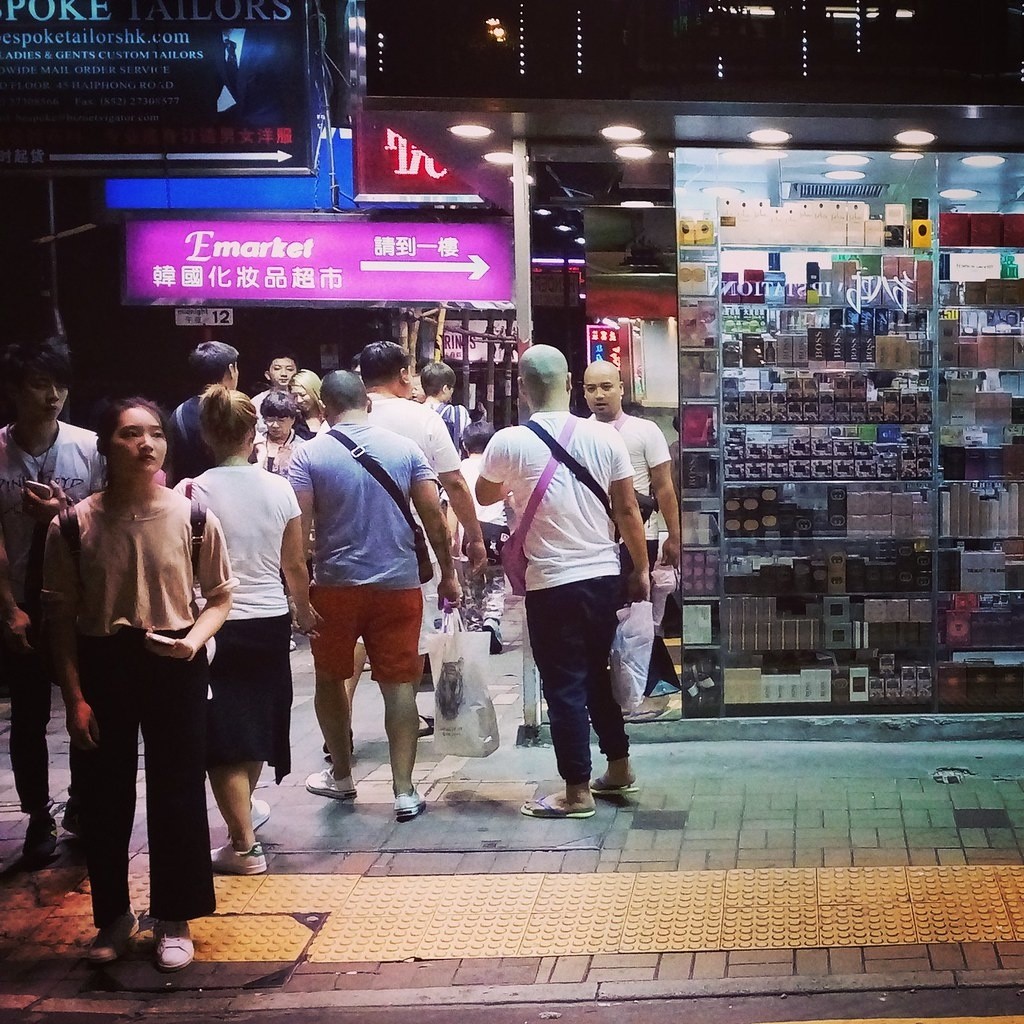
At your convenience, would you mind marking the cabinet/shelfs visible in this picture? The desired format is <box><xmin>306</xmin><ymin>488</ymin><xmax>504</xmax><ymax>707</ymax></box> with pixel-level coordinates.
<box><xmin>580</xmin><ymin>190</ymin><xmax>1024</xmax><ymax>717</ymax></box>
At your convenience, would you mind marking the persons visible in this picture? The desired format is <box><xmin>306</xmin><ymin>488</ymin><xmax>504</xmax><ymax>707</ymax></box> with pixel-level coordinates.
<box><xmin>256</xmin><ymin>392</ymin><xmax>306</xmax><ymax>479</ymax></box>
<box><xmin>290</xmin><ymin>370</ymin><xmax>460</xmax><ymax>817</ymax></box>
<box><xmin>441</xmin><ymin>422</ymin><xmax>516</xmax><ymax>654</ymax></box>
<box><xmin>476</xmin><ymin>399</ymin><xmax>488</xmax><ymax>422</ymax></box>
<box><xmin>287</xmin><ymin>369</ymin><xmax>325</xmax><ymax>441</ymax></box>
<box><xmin>317</xmin><ymin>341</ymin><xmax>488</xmax><ymax>754</ymax></box>
<box><xmin>0</xmin><ymin>336</ymin><xmax>109</xmax><ymax>858</ymax></box>
<box><xmin>476</xmin><ymin>344</ymin><xmax>650</xmax><ymax>818</ymax></box>
<box><xmin>250</xmin><ymin>353</ymin><xmax>298</xmax><ymax>417</ymax></box>
<box><xmin>422</xmin><ymin>363</ymin><xmax>472</xmax><ymax>460</ymax></box>
<box><xmin>44</xmin><ymin>397</ymin><xmax>240</xmax><ymax>972</ymax></box>
<box><xmin>411</xmin><ymin>373</ymin><xmax>427</xmax><ymax>404</ymax></box>
<box><xmin>164</xmin><ymin>341</ymin><xmax>239</xmax><ymax>482</ymax></box>
<box><xmin>174</xmin><ymin>384</ymin><xmax>315</xmax><ymax>874</ymax></box>
<box><xmin>583</xmin><ymin>360</ymin><xmax>680</xmax><ymax>720</ymax></box>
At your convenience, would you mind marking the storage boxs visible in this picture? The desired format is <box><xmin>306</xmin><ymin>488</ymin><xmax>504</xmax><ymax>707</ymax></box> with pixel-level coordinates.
<box><xmin>847</xmin><ymin>491</ymin><xmax>870</xmax><ymax>514</ymax></box>
<box><xmin>891</xmin><ymin>492</ymin><xmax>912</xmax><ymax>516</ymax></box>
<box><xmin>959</xmin><ymin>342</ymin><xmax>978</xmax><ymax>367</ymax></box>
<box><xmin>886</xmin><ymin>599</ymin><xmax>909</xmax><ymax>622</ymax></box>
<box><xmin>864</xmin><ymin>599</ymin><xmax>885</xmax><ymax>623</ymax></box>
<box><xmin>868</xmin><ymin>514</ymin><xmax>892</xmax><ymax>536</ymax></box>
<box><xmin>808</xmin><ymin>327</ymin><xmax>826</xmax><ymax>360</ymax></box>
<box><xmin>892</xmin><ymin>515</ymin><xmax>913</xmax><ymax>536</ymax></box>
<box><xmin>846</xmin><ymin>557</ymin><xmax>865</xmax><ymax>593</ymax></box>
<box><xmin>939</xmin><ymin>343</ymin><xmax>959</xmax><ymax>367</ymax></box>
<box><xmin>948</xmin><ymin>379</ymin><xmax>975</xmax><ymax>401</ymax></box>
<box><xmin>792</xmin><ymin>334</ymin><xmax>808</xmax><ymax>367</ymax></box>
<box><xmin>939</xmin><ymin>319</ymin><xmax>958</xmax><ymax>342</ymax></box>
<box><xmin>977</xmin><ymin>335</ymin><xmax>995</xmax><ymax>368</ymax></box>
<box><xmin>909</xmin><ymin>599</ymin><xmax>932</xmax><ymax>623</ymax></box>
<box><xmin>870</xmin><ymin>491</ymin><xmax>892</xmax><ymax>515</ymax></box>
<box><xmin>995</xmin><ymin>335</ymin><xmax>1014</xmax><ymax>369</ymax></box>
<box><xmin>846</xmin><ymin>514</ymin><xmax>868</xmax><ymax>535</ymax></box>
<box><xmin>776</xmin><ymin>334</ymin><xmax>793</xmax><ymax>367</ymax></box>
<box><xmin>949</xmin><ymin>402</ymin><xmax>975</xmax><ymax>425</ymax></box>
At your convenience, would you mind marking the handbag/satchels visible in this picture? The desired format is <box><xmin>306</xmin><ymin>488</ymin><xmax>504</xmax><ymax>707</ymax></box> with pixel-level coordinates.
<box><xmin>413</xmin><ymin>525</ymin><xmax>434</xmax><ymax>584</ymax></box>
<box><xmin>462</xmin><ymin>519</ymin><xmax>511</xmax><ymax>566</ymax></box>
<box><xmin>0</xmin><ymin>601</ymin><xmax>44</xmax><ymax>698</ymax></box>
<box><xmin>613</xmin><ymin>601</ymin><xmax>654</xmax><ymax>709</ymax></box>
<box><xmin>499</xmin><ymin>532</ymin><xmax>528</xmax><ymax>596</ymax></box>
<box><xmin>631</xmin><ymin>489</ymin><xmax>659</xmax><ymax>524</ymax></box>
<box><xmin>426</xmin><ymin>606</ymin><xmax>500</xmax><ymax>758</ymax></box>
<box><xmin>650</xmin><ymin>563</ymin><xmax>680</xmax><ymax>626</ymax></box>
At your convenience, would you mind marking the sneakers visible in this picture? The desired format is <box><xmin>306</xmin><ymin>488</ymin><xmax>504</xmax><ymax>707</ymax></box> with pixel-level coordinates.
<box><xmin>152</xmin><ymin>917</ymin><xmax>194</xmax><ymax>971</ymax></box>
<box><xmin>210</xmin><ymin>838</ymin><xmax>267</xmax><ymax>874</ymax></box>
<box><xmin>394</xmin><ymin>783</ymin><xmax>426</xmax><ymax>816</ymax></box>
<box><xmin>245</xmin><ymin>796</ymin><xmax>270</xmax><ymax>831</ymax></box>
<box><xmin>482</xmin><ymin>617</ymin><xmax>503</xmax><ymax>653</ymax></box>
<box><xmin>305</xmin><ymin>769</ymin><xmax>357</xmax><ymax>799</ymax></box>
<box><xmin>88</xmin><ymin>903</ymin><xmax>140</xmax><ymax>963</ymax></box>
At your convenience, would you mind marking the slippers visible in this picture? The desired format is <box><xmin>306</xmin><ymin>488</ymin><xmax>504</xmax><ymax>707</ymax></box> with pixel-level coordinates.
<box><xmin>588</xmin><ymin>777</ymin><xmax>639</xmax><ymax>794</ymax></box>
<box><xmin>624</xmin><ymin>703</ymin><xmax>671</xmax><ymax>722</ymax></box>
<box><xmin>520</xmin><ymin>795</ymin><xmax>595</xmax><ymax>818</ymax></box>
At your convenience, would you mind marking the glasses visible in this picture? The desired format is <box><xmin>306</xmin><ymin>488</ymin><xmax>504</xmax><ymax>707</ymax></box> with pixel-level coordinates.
<box><xmin>264</xmin><ymin>417</ymin><xmax>289</xmax><ymax>426</ymax></box>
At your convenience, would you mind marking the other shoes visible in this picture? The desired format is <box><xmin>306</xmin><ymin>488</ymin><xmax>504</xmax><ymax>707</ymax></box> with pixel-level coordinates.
<box><xmin>23</xmin><ymin>812</ymin><xmax>57</xmax><ymax>860</ymax></box>
<box><xmin>60</xmin><ymin>785</ymin><xmax>86</xmax><ymax>839</ymax></box>
<box><xmin>417</xmin><ymin>715</ymin><xmax>434</xmax><ymax>737</ymax></box>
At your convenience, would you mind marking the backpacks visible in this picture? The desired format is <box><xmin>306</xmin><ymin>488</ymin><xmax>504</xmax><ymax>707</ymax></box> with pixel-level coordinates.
<box><xmin>437</xmin><ymin>404</ymin><xmax>468</xmax><ymax>460</ymax></box>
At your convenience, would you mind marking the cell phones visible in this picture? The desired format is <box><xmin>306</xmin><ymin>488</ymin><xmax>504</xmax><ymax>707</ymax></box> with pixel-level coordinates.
<box><xmin>23</xmin><ymin>481</ymin><xmax>53</xmax><ymax>500</ymax></box>
<box><xmin>145</xmin><ymin>633</ymin><xmax>177</xmax><ymax>647</ymax></box>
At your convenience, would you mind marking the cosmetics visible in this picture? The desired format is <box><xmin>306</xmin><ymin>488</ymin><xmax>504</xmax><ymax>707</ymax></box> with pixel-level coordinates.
<box><xmin>678</xmin><ymin>192</ymin><xmax>1024</xmax><ymax>718</ymax></box>
